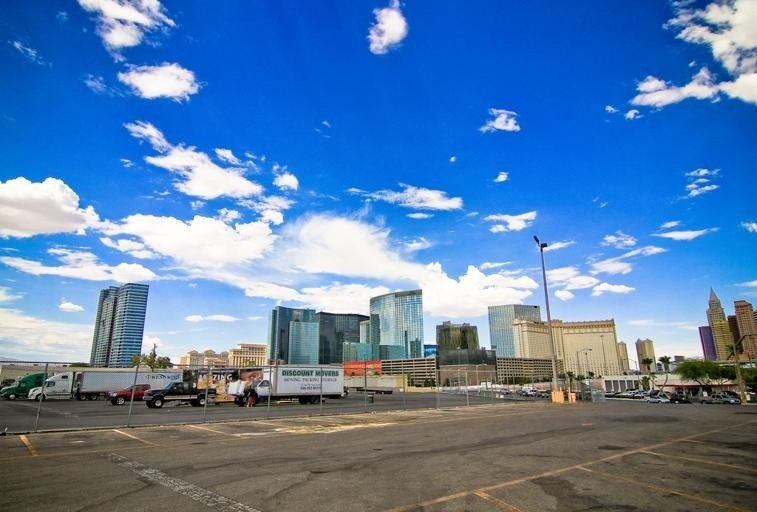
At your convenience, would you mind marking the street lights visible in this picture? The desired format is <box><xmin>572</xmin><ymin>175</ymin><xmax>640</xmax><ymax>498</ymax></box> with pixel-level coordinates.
<box><xmin>533</xmin><ymin>235</ymin><xmax>559</xmax><ymax>391</ymax></box>
<box><xmin>457</xmin><ymin>347</ymin><xmax>461</xmax><ymax>393</ymax></box>
<box><xmin>576</xmin><ymin>348</ymin><xmax>592</xmax><ymax>380</ymax></box>
<box><xmin>476</xmin><ymin>364</ymin><xmax>487</xmax><ymax>391</ymax></box>
<box><xmin>623</xmin><ymin>358</ymin><xmax>639</xmax><ymax>385</ymax></box>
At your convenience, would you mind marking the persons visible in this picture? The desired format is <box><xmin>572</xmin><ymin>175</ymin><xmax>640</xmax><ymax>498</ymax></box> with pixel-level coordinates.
<box><xmin>246</xmin><ymin>389</ymin><xmax>255</xmax><ymax>408</ymax></box>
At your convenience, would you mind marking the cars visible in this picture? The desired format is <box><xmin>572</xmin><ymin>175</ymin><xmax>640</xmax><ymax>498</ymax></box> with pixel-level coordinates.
<box><xmin>500</xmin><ymin>387</ymin><xmax>692</xmax><ymax>404</ymax></box>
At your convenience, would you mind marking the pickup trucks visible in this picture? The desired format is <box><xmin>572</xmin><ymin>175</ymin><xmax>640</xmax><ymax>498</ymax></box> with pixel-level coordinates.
<box><xmin>700</xmin><ymin>390</ymin><xmax>741</xmax><ymax>404</ymax></box>
<box><xmin>108</xmin><ymin>381</ymin><xmax>217</xmax><ymax>407</ymax></box>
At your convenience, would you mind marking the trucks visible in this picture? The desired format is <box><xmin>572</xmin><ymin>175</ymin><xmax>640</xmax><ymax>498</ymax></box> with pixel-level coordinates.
<box><xmin>1</xmin><ymin>369</ymin><xmax>182</xmax><ymax>402</ymax></box>
<box><xmin>228</xmin><ymin>364</ymin><xmax>344</xmax><ymax>404</ymax></box>
